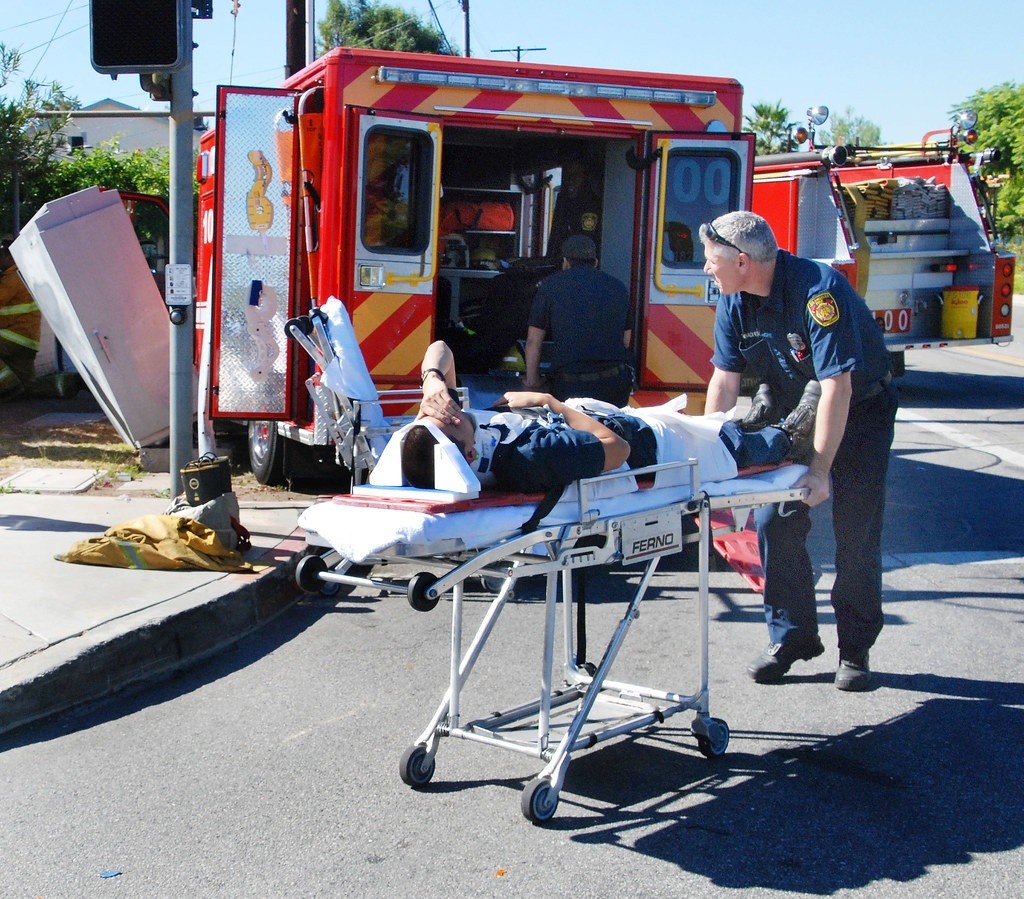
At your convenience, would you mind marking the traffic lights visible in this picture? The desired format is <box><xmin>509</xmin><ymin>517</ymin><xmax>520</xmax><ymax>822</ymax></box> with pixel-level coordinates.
<box><xmin>89</xmin><ymin>0</ymin><xmax>185</xmax><ymax>81</ymax></box>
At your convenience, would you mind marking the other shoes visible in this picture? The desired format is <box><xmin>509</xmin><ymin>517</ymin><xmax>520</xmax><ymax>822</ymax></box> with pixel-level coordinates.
<box><xmin>776</xmin><ymin>379</ymin><xmax>822</xmax><ymax>460</ymax></box>
<box><xmin>740</xmin><ymin>383</ymin><xmax>780</xmax><ymax>430</ymax></box>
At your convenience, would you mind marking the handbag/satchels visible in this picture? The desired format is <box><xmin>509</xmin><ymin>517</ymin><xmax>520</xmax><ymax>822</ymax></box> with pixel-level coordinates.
<box><xmin>437</xmin><ymin>200</ymin><xmax>514</xmax><ymax>234</ymax></box>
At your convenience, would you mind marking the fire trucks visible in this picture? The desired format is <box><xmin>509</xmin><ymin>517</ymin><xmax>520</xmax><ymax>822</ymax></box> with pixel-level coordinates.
<box><xmin>752</xmin><ymin>106</ymin><xmax>1016</xmax><ymax>377</ymax></box>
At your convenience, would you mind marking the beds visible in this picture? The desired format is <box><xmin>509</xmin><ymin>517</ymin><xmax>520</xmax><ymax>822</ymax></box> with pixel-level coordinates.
<box><xmin>297</xmin><ymin>463</ymin><xmax>821</xmax><ymax>807</ymax></box>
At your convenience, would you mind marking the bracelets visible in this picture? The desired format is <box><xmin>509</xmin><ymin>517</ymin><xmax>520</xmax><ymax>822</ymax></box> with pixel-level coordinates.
<box><xmin>418</xmin><ymin>368</ymin><xmax>447</xmax><ymax>383</ymax></box>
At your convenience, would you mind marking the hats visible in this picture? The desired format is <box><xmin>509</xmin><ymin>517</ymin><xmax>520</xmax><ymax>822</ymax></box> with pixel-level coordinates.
<box><xmin>560</xmin><ymin>234</ymin><xmax>596</xmax><ymax>260</ymax></box>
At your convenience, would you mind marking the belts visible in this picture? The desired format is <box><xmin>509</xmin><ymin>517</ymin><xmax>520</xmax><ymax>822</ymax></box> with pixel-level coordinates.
<box><xmin>849</xmin><ymin>372</ymin><xmax>892</xmax><ymax>408</ymax></box>
<box><xmin>582</xmin><ymin>407</ymin><xmax>633</xmax><ymax>443</ymax></box>
<box><xmin>551</xmin><ymin>363</ymin><xmax>626</xmax><ymax>381</ymax></box>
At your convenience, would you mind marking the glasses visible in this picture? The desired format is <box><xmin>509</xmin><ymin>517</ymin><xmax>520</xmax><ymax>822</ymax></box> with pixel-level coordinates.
<box><xmin>706</xmin><ymin>217</ymin><xmax>742</xmax><ymax>253</ymax></box>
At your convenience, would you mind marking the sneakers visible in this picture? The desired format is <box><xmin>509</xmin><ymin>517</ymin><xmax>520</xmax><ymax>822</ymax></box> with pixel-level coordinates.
<box><xmin>835</xmin><ymin>648</ymin><xmax>871</xmax><ymax>691</ymax></box>
<box><xmin>748</xmin><ymin>633</ymin><xmax>824</xmax><ymax>683</ymax></box>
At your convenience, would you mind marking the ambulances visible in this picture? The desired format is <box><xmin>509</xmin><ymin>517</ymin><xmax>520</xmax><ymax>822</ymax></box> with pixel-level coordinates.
<box><xmin>53</xmin><ymin>46</ymin><xmax>765</xmax><ymax>596</ymax></box>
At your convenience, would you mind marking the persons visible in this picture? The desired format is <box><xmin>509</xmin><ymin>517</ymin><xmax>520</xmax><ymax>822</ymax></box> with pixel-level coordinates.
<box><xmin>402</xmin><ymin>340</ymin><xmax>822</xmax><ymax>493</ymax></box>
<box><xmin>522</xmin><ymin>235</ymin><xmax>636</xmax><ymax>408</ymax></box>
<box><xmin>699</xmin><ymin>211</ymin><xmax>899</xmax><ymax>690</ymax></box>
<box><xmin>547</xmin><ymin>145</ymin><xmax>602</xmax><ymax>268</ymax></box>
<box><xmin>0</xmin><ymin>234</ymin><xmax>44</xmax><ymax>396</ymax></box>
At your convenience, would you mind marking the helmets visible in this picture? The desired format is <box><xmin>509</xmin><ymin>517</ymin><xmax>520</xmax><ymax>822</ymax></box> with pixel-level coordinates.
<box><xmin>471</xmin><ymin>248</ymin><xmax>497</xmax><ymax>270</ymax></box>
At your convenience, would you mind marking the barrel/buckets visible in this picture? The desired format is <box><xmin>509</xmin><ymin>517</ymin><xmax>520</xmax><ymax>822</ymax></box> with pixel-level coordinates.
<box><xmin>938</xmin><ymin>286</ymin><xmax>982</xmax><ymax>339</ymax></box>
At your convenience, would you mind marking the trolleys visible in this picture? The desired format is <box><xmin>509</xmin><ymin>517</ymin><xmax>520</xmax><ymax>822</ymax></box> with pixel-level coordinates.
<box><xmin>294</xmin><ymin>457</ymin><xmax>813</xmax><ymax>823</ymax></box>
